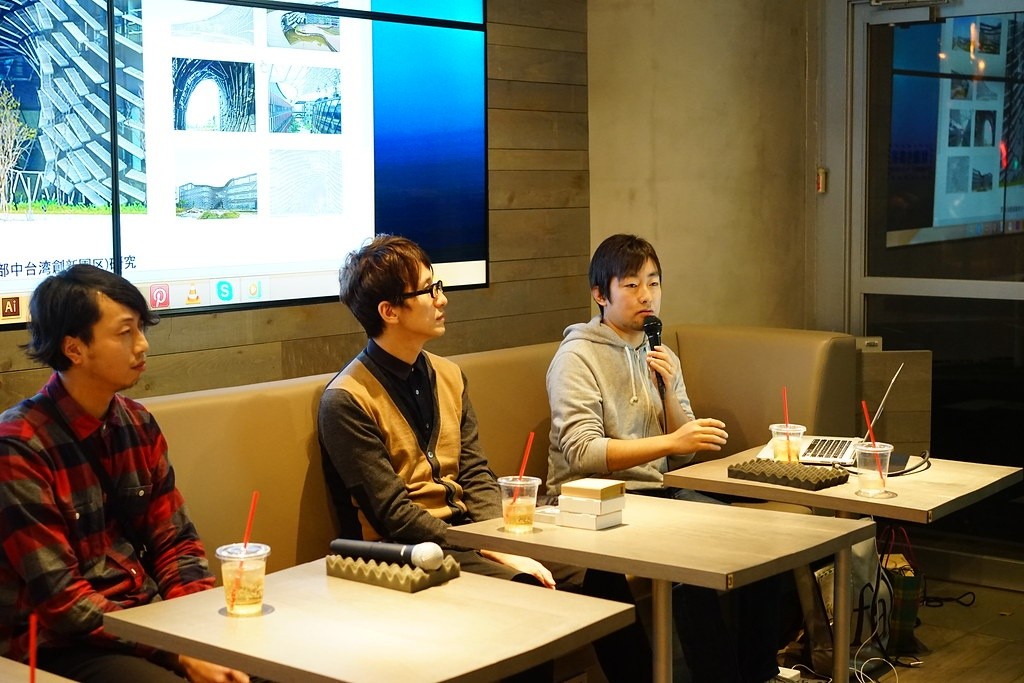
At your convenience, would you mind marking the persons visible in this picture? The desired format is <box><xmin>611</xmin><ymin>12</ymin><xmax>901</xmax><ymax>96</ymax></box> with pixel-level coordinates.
<box><xmin>1</xmin><ymin>263</ymin><xmax>274</xmax><ymax>683</ymax></box>
<box><xmin>316</xmin><ymin>232</ymin><xmax>647</xmax><ymax>683</ymax></box>
<box><xmin>545</xmin><ymin>234</ymin><xmax>807</xmax><ymax>683</ymax></box>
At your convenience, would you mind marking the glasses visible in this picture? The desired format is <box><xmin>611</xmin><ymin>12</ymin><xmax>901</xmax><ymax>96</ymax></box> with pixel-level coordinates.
<box><xmin>399</xmin><ymin>280</ymin><xmax>444</xmax><ymax>299</ymax></box>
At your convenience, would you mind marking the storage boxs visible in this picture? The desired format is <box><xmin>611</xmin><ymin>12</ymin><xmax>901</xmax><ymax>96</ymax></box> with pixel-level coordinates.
<box><xmin>555</xmin><ymin>510</ymin><xmax>623</xmax><ymax>531</ymax></box>
<box><xmin>560</xmin><ymin>477</ymin><xmax>626</xmax><ymax>502</ymax></box>
<box><xmin>557</xmin><ymin>495</ymin><xmax>625</xmax><ymax>515</ymax></box>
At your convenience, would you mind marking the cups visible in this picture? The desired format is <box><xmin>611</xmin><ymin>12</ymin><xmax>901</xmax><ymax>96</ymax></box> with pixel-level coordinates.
<box><xmin>855</xmin><ymin>441</ymin><xmax>894</xmax><ymax>495</ymax></box>
<box><xmin>769</xmin><ymin>423</ymin><xmax>806</xmax><ymax>462</ymax></box>
<box><xmin>497</xmin><ymin>475</ymin><xmax>542</xmax><ymax>533</ymax></box>
<box><xmin>214</xmin><ymin>542</ymin><xmax>272</xmax><ymax>618</ymax></box>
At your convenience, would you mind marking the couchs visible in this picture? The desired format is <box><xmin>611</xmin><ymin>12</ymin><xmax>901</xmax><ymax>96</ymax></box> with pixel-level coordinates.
<box><xmin>0</xmin><ymin>322</ymin><xmax>858</xmax><ymax>683</ymax></box>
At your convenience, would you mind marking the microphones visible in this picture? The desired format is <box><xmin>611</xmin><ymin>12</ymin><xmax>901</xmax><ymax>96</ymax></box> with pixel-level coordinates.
<box><xmin>330</xmin><ymin>540</ymin><xmax>444</xmax><ymax>570</ymax></box>
<box><xmin>644</xmin><ymin>315</ymin><xmax>666</xmax><ymax>400</ymax></box>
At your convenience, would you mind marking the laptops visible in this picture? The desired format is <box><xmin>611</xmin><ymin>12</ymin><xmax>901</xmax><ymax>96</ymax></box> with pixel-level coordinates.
<box><xmin>757</xmin><ymin>362</ymin><xmax>904</xmax><ymax>466</ymax></box>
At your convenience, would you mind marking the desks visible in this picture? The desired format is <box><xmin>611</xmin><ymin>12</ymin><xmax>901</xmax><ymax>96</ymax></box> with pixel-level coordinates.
<box><xmin>103</xmin><ymin>557</ymin><xmax>637</xmax><ymax>683</ymax></box>
<box><xmin>443</xmin><ymin>493</ymin><xmax>875</xmax><ymax>683</ymax></box>
<box><xmin>663</xmin><ymin>436</ymin><xmax>1024</xmax><ymax>683</ymax></box>
